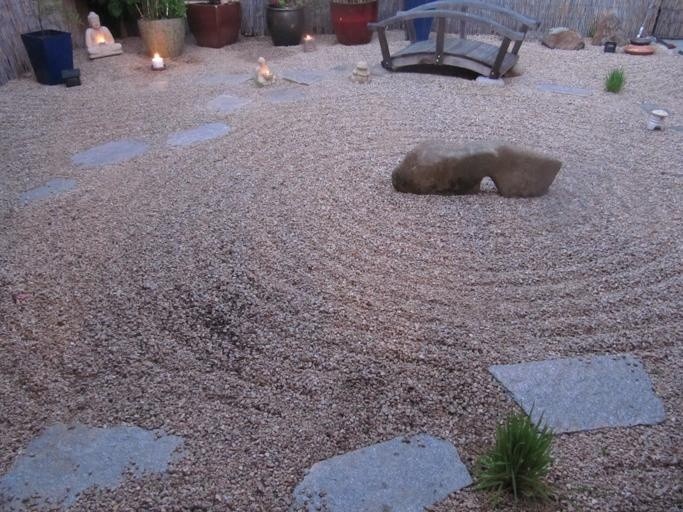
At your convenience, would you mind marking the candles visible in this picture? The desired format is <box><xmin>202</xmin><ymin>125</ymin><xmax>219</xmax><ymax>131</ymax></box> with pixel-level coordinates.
<box><xmin>152</xmin><ymin>52</ymin><xmax>163</xmax><ymax>67</ymax></box>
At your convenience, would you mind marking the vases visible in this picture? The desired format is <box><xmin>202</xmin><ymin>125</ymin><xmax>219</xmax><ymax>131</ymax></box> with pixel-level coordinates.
<box><xmin>330</xmin><ymin>0</ymin><xmax>378</xmax><ymax>45</ymax></box>
<box><xmin>186</xmin><ymin>2</ymin><xmax>241</xmax><ymax>49</ymax></box>
<box><xmin>404</xmin><ymin>0</ymin><xmax>437</xmax><ymax>42</ymax></box>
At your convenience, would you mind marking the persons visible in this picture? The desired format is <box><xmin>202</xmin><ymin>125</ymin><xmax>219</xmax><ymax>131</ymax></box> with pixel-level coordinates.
<box><xmin>85</xmin><ymin>10</ymin><xmax>122</xmax><ymax>60</ymax></box>
<box><xmin>253</xmin><ymin>56</ymin><xmax>277</xmax><ymax>86</ymax></box>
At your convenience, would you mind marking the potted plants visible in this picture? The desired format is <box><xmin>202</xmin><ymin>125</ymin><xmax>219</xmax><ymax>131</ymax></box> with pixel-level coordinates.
<box><xmin>267</xmin><ymin>0</ymin><xmax>305</xmax><ymax>46</ymax></box>
<box><xmin>128</xmin><ymin>0</ymin><xmax>189</xmax><ymax>58</ymax></box>
<box><xmin>21</xmin><ymin>0</ymin><xmax>87</xmax><ymax>85</ymax></box>
